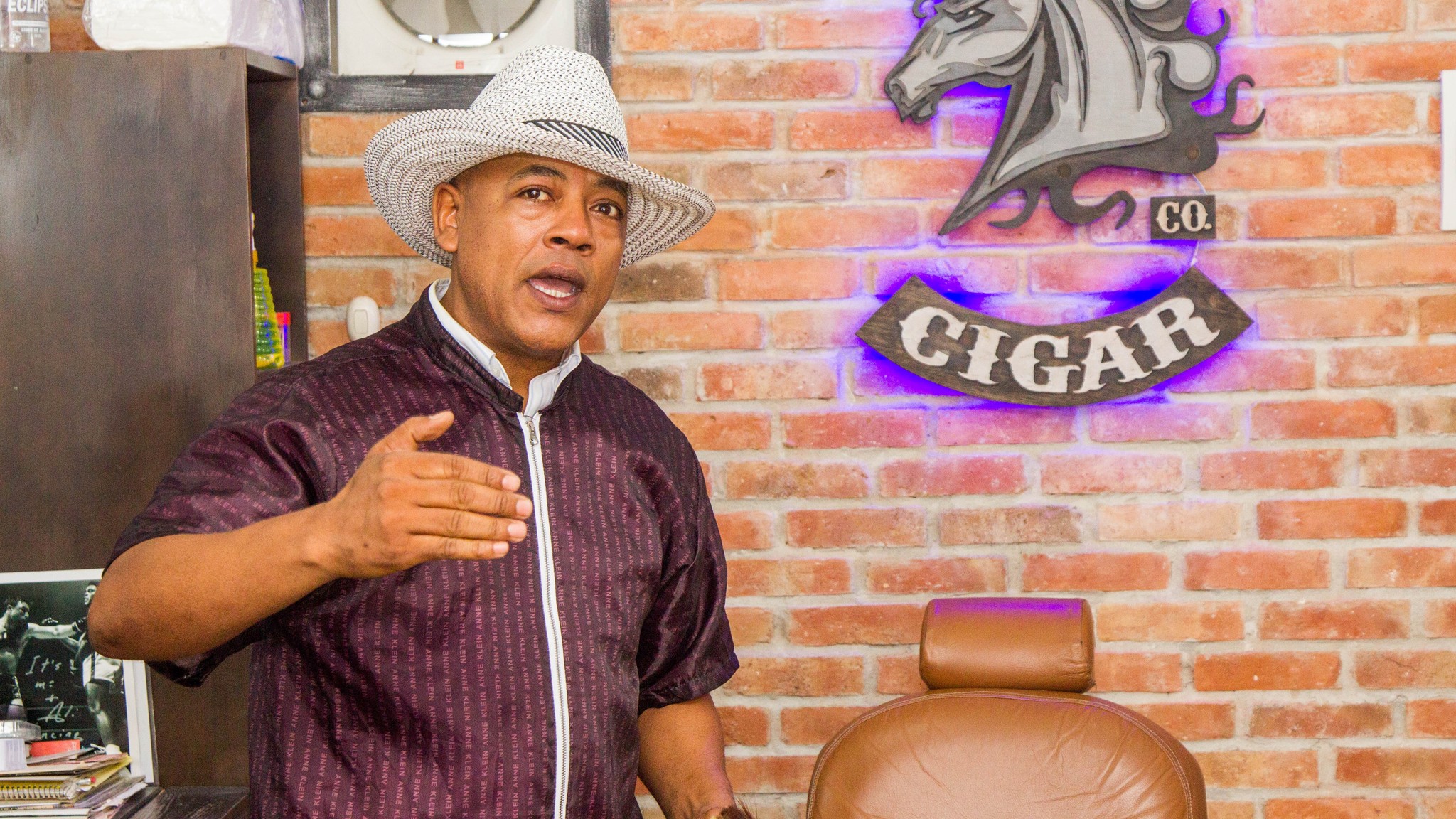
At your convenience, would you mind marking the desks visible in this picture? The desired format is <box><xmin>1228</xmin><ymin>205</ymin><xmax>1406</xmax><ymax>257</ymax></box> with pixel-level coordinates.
<box><xmin>122</xmin><ymin>786</ymin><xmax>252</xmax><ymax>819</ymax></box>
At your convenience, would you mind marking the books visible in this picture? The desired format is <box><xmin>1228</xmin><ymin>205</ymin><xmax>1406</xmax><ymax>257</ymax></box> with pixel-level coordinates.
<box><xmin>0</xmin><ymin>748</ymin><xmax>148</xmax><ymax>819</ymax></box>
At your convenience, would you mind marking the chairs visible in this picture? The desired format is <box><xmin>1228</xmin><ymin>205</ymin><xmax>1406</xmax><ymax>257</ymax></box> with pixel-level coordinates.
<box><xmin>806</xmin><ymin>595</ymin><xmax>1212</xmax><ymax>819</ymax></box>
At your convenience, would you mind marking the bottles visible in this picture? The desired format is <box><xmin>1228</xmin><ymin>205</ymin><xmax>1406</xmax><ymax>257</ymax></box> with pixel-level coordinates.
<box><xmin>276</xmin><ymin>313</ymin><xmax>291</xmax><ymax>362</ymax></box>
<box><xmin>251</xmin><ymin>212</ymin><xmax>285</xmax><ymax>369</ymax></box>
<box><xmin>0</xmin><ymin>0</ymin><xmax>51</xmax><ymax>53</ymax></box>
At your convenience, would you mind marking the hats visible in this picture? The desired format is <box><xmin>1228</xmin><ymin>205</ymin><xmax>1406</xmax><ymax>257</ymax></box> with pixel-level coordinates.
<box><xmin>364</xmin><ymin>46</ymin><xmax>719</xmax><ymax>268</ymax></box>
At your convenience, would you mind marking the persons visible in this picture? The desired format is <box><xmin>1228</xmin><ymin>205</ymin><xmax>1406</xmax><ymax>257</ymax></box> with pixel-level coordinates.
<box><xmin>87</xmin><ymin>47</ymin><xmax>756</xmax><ymax>819</ymax></box>
<box><xmin>0</xmin><ymin>595</ymin><xmax>88</xmax><ymax>721</ymax></box>
<box><xmin>43</xmin><ymin>582</ymin><xmax>123</xmax><ymax>754</ymax></box>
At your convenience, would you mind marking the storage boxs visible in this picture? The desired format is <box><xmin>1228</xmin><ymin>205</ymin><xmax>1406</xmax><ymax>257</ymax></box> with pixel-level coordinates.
<box><xmin>0</xmin><ymin>719</ymin><xmax>43</xmax><ymax>743</ymax></box>
<box><xmin>0</xmin><ymin>738</ymin><xmax>27</xmax><ymax>772</ymax></box>
<box><xmin>30</xmin><ymin>739</ymin><xmax>80</xmax><ymax>757</ymax></box>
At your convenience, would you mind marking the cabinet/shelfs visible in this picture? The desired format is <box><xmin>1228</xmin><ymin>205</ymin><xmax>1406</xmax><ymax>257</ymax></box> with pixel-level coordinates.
<box><xmin>0</xmin><ymin>44</ymin><xmax>307</xmax><ymax>818</ymax></box>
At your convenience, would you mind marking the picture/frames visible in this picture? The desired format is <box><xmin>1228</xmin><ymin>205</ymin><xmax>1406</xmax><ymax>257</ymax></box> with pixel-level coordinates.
<box><xmin>0</xmin><ymin>568</ymin><xmax>160</xmax><ymax>785</ymax></box>
